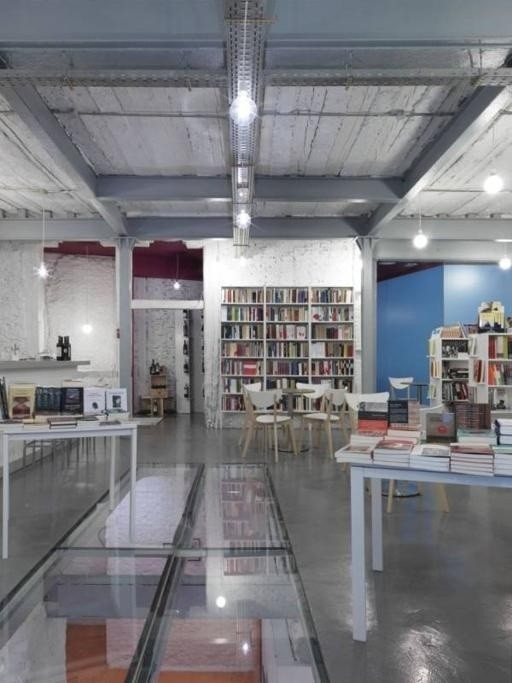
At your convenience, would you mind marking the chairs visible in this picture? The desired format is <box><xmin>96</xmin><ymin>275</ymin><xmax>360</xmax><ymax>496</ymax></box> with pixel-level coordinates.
<box><xmin>240</xmin><ymin>373</ymin><xmax>414</xmax><ymax>465</ymax></box>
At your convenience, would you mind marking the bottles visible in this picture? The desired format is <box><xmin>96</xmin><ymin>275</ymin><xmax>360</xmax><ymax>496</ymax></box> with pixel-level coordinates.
<box><xmin>183</xmin><ymin>309</ymin><xmax>188</xmax><ymax>318</ymax></box>
<box><xmin>184</xmin><ymin>383</ymin><xmax>189</xmax><ymax>398</ymax></box>
<box><xmin>56</xmin><ymin>336</ymin><xmax>71</xmax><ymax>361</ymax></box>
<box><xmin>184</xmin><ymin>359</ymin><xmax>189</xmax><ymax>373</ymax></box>
<box><xmin>183</xmin><ymin>320</ymin><xmax>188</xmax><ymax>336</ymax></box>
<box><xmin>183</xmin><ymin>340</ymin><xmax>188</xmax><ymax>355</ymax></box>
<box><xmin>150</xmin><ymin>359</ymin><xmax>160</xmax><ymax>375</ymax></box>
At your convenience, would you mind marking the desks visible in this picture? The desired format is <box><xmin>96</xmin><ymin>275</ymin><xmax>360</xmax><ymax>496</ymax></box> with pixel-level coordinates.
<box><xmin>0</xmin><ymin>417</ymin><xmax>141</xmax><ymax>558</ymax></box>
<box><xmin>335</xmin><ymin>440</ymin><xmax>512</xmax><ymax>644</ymax></box>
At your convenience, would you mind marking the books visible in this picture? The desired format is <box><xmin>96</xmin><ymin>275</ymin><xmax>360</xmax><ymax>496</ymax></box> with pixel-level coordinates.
<box><xmin>488</xmin><ymin>335</ymin><xmax>512</xmax><ymax>359</ymax></box>
<box><xmin>220</xmin><ymin>466</ymin><xmax>287</xmax><ymax>576</ymax></box>
<box><xmin>49</xmin><ymin>417</ymin><xmax>77</xmax><ymax>429</ymax></box>
<box><xmin>221</xmin><ymin>287</ymin><xmax>354</xmax><ymax>357</ymax></box>
<box><xmin>221</xmin><ymin>358</ymin><xmax>310</xmax><ymax>411</ymax></box>
<box><xmin>35</xmin><ymin>387</ymin><xmax>83</xmax><ymax>415</ymax></box>
<box><xmin>442</xmin><ymin>340</ymin><xmax>468</xmax><ymax>358</ymax></box>
<box><xmin>310</xmin><ymin>360</ymin><xmax>512</xmax><ymax>479</ymax></box>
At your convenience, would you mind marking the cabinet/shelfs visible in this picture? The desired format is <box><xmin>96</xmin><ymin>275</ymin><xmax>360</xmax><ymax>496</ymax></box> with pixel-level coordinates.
<box><xmin>428</xmin><ymin>330</ymin><xmax>511</xmax><ymax>421</ymax></box>
<box><xmin>217</xmin><ymin>283</ymin><xmax>356</xmax><ymax>417</ymax></box>
